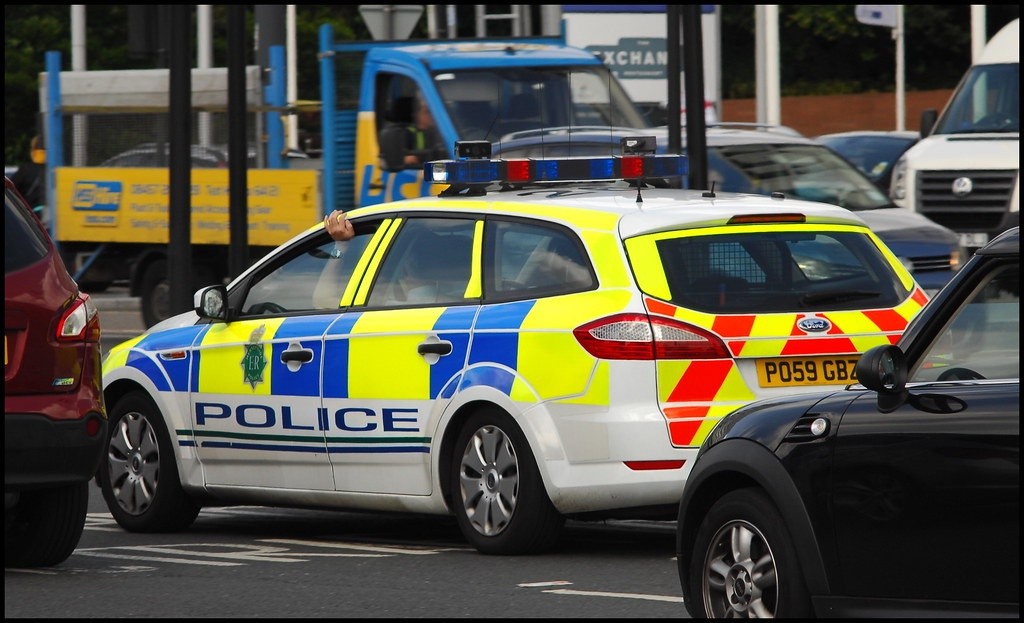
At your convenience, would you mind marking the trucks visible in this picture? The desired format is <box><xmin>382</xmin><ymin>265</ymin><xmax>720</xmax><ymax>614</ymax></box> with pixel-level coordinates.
<box><xmin>45</xmin><ymin>16</ymin><xmax>656</xmax><ymax>332</ymax></box>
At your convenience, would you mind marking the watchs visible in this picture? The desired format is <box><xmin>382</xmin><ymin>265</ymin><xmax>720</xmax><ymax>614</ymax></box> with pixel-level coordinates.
<box><xmin>329</xmin><ymin>249</ymin><xmax>345</xmax><ymax>259</ymax></box>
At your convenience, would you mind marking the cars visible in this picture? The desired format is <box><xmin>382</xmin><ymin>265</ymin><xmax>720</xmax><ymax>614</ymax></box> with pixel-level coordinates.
<box><xmin>817</xmin><ymin>127</ymin><xmax>923</xmax><ymax>196</ymax></box>
<box><xmin>4</xmin><ymin>142</ymin><xmax>309</xmax><ymax>293</ymax></box>
<box><xmin>676</xmin><ymin>226</ymin><xmax>1021</xmax><ymax>621</ymax></box>
<box><xmin>91</xmin><ymin>153</ymin><xmax>932</xmax><ymax>557</ymax></box>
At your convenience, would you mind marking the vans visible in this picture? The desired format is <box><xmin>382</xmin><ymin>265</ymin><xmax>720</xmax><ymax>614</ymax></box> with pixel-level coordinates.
<box><xmin>885</xmin><ymin>18</ymin><xmax>1022</xmax><ymax>253</ymax></box>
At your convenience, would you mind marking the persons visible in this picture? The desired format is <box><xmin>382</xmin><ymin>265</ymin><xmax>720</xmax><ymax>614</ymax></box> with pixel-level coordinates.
<box><xmin>313</xmin><ymin>209</ymin><xmax>460</xmax><ymax>309</ymax></box>
<box><xmin>378</xmin><ymin>92</ymin><xmax>445</xmax><ymax>168</ymax></box>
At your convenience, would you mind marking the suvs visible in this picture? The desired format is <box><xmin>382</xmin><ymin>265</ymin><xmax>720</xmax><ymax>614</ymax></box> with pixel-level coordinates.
<box><xmin>0</xmin><ymin>170</ymin><xmax>108</xmax><ymax>573</ymax></box>
<box><xmin>490</xmin><ymin>122</ymin><xmax>968</xmax><ymax>302</ymax></box>
